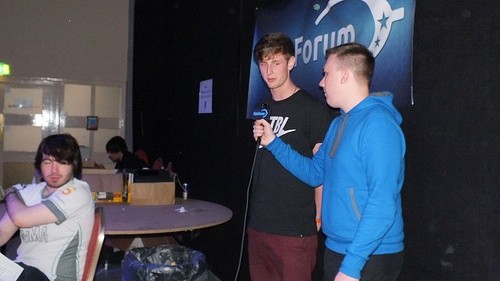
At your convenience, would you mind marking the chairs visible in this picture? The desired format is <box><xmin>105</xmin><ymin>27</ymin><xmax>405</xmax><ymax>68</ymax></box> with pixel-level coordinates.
<box><xmin>81</xmin><ymin>213</ymin><xmax>105</xmax><ymax>281</ymax></box>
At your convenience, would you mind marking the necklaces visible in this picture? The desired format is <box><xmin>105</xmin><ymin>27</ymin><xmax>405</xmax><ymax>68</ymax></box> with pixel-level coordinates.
<box><xmin>294</xmin><ymin>86</ymin><xmax>297</xmax><ymax>93</ymax></box>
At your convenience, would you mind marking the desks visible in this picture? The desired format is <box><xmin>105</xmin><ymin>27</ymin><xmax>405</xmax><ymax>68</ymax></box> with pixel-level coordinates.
<box><xmin>103</xmin><ymin>198</ymin><xmax>233</xmax><ymax>250</ymax></box>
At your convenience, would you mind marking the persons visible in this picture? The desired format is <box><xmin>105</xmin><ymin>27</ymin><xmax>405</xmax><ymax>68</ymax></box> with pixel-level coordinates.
<box><xmin>94</xmin><ymin>136</ymin><xmax>151</xmax><ymax>181</ymax></box>
<box><xmin>0</xmin><ymin>133</ymin><xmax>96</xmax><ymax>281</ymax></box>
<box><xmin>247</xmin><ymin>31</ymin><xmax>332</xmax><ymax>281</ymax></box>
<box><xmin>253</xmin><ymin>42</ymin><xmax>405</xmax><ymax>281</ymax></box>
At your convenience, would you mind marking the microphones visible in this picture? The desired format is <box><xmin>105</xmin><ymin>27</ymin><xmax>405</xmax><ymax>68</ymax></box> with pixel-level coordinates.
<box><xmin>257</xmin><ymin>103</ymin><xmax>271</xmax><ymax>145</ymax></box>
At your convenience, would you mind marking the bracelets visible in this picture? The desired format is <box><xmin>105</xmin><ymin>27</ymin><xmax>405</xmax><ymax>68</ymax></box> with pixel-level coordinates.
<box><xmin>315</xmin><ymin>220</ymin><xmax>321</xmax><ymax>222</ymax></box>
<box><xmin>5</xmin><ymin>191</ymin><xmax>14</xmax><ymax>199</ymax></box>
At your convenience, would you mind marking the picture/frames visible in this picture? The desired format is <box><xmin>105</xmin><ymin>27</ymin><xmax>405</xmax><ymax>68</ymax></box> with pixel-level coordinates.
<box><xmin>87</xmin><ymin>116</ymin><xmax>98</xmax><ymax>130</ymax></box>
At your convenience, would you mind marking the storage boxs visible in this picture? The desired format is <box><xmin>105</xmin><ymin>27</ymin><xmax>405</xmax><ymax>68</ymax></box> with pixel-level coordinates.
<box><xmin>81</xmin><ymin>173</ymin><xmax>123</xmax><ymax>206</ymax></box>
<box><xmin>126</xmin><ymin>172</ymin><xmax>176</xmax><ymax>205</ymax></box>
